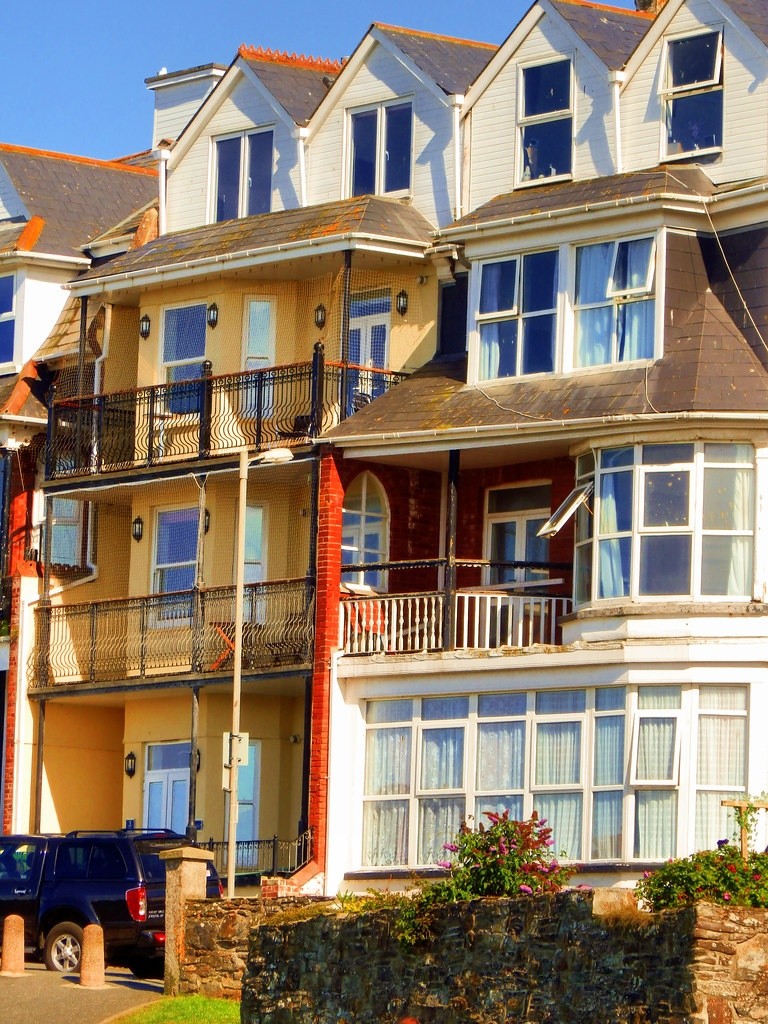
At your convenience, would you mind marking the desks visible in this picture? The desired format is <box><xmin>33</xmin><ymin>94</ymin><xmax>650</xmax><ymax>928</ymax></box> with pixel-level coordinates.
<box><xmin>207</xmin><ymin>621</ymin><xmax>266</xmax><ymax>671</ymax></box>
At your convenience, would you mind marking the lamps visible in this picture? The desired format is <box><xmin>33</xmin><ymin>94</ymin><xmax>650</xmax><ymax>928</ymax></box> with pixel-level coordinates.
<box><xmin>189</xmin><ymin>749</ymin><xmax>200</xmax><ymax>772</ymax></box>
<box><xmin>206</xmin><ymin>303</ymin><xmax>219</xmax><ymax>330</ymax></box>
<box><xmin>139</xmin><ymin>314</ymin><xmax>151</xmax><ymax>341</ymax></box>
<box><xmin>396</xmin><ymin>290</ymin><xmax>408</xmax><ymax>317</ymax></box>
<box><xmin>199</xmin><ymin>508</ymin><xmax>210</xmax><ymax>535</ymax></box>
<box><xmin>315</xmin><ymin>303</ymin><xmax>326</xmax><ymax>330</ymax></box>
<box><xmin>133</xmin><ymin>516</ymin><xmax>144</xmax><ymax>543</ymax></box>
<box><xmin>124</xmin><ymin>751</ymin><xmax>136</xmax><ymax>779</ymax></box>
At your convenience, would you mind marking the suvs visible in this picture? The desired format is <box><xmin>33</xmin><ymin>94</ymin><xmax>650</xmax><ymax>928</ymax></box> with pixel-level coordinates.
<box><xmin>0</xmin><ymin>826</ymin><xmax>226</xmax><ymax>975</ymax></box>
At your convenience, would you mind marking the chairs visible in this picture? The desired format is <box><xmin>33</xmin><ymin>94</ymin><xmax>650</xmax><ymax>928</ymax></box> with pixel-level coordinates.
<box><xmin>0</xmin><ymin>848</ymin><xmax>128</xmax><ymax>886</ymax></box>
<box><xmin>266</xmin><ymin>613</ymin><xmax>309</xmax><ymax>667</ymax></box>
<box><xmin>275</xmin><ymin>415</ymin><xmax>313</xmax><ymax>441</ymax></box>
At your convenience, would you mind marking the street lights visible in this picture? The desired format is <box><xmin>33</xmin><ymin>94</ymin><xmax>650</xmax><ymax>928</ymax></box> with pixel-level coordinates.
<box><xmin>225</xmin><ymin>445</ymin><xmax>296</xmax><ymax>900</ymax></box>
